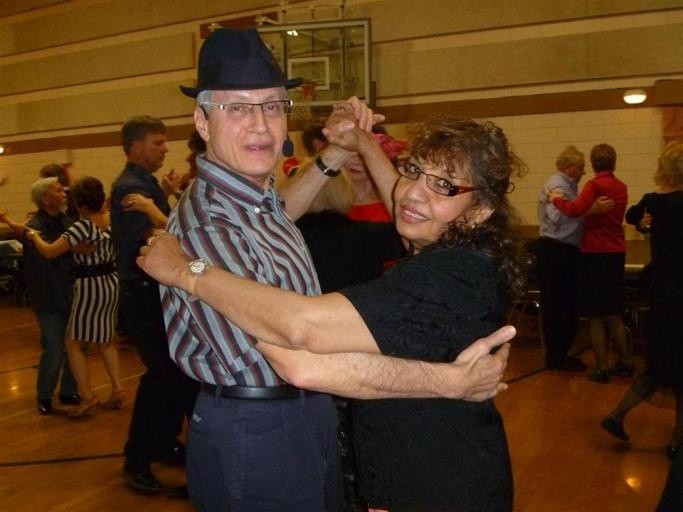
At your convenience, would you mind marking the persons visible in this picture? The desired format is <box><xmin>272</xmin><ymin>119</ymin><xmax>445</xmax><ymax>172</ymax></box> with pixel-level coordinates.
<box><xmin>134</xmin><ymin>113</ymin><xmax>532</xmax><ymax>512</ymax></box>
<box><xmin>533</xmin><ymin>145</ymin><xmax>614</xmax><ymax>371</ymax></box>
<box><xmin>598</xmin><ymin>142</ymin><xmax>681</xmax><ymax>461</ymax></box>
<box><xmin>156</xmin><ymin>24</ymin><xmax>520</xmax><ymax>512</ymax></box>
<box><xmin>109</xmin><ymin>112</ymin><xmax>195</xmax><ymax>497</ymax></box>
<box><xmin>635</xmin><ymin>204</ymin><xmax>652</xmax><ymax>233</ymax></box>
<box><xmin>0</xmin><ymin>106</ymin><xmax>408</xmax><ymax>421</ymax></box>
<box><xmin>544</xmin><ymin>143</ymin><xmax>634</xmax><ymax>383</ymax></box>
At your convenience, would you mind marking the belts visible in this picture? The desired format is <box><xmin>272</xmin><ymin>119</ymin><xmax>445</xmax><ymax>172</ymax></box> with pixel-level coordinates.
<box><xmin>201</xmin><ymin>384</ymin><xmax>320</xmax><ymax>400</ymax></box>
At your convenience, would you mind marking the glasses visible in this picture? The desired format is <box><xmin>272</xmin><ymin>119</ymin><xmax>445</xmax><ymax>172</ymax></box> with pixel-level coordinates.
<box><xmin>397</xmin><ymin>156</ymin><xmax>480</xmax><ymax>196</ymax></box>
<box><xmin>199</xmin><ymin>100</ymin><xmax>292</xmax><ymax>119</ymax></box>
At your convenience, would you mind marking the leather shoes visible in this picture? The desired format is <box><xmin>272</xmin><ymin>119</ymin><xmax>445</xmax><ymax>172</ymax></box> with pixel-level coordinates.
<box><xmin>122</xmin><ymin>463</ymin><xmax>163</xmax><ymax>495</ymax></box>
<box><xmin>546</xmin><ymin>360</ymin><xmax>586</xmax><ymax>371</ymax></box>
<box><xmin>36</xmin><ymin>397</ymin><xmax>51</xmax><ymax>414</ymax></box>
<box><xmin>59</xmin><ymin>392</ymin><xmax>80</xmax><ymax>404</ymax></box>
<box><xmin>601</xmin><ymin>416</ymin><xmax>630</xmax><ymax>441</ymax></box>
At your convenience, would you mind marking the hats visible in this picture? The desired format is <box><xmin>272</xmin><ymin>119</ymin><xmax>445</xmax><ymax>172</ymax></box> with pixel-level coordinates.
<box><xmin>179</xmin><ymin>26</ymin><xmax>303</xmax><ymax>98</ymax></box>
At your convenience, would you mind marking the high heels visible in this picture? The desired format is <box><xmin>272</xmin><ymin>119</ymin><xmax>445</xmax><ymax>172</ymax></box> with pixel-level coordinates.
<box><xmin>67</xmin><ymin>395</ymin><xmax>99</xmax><ymax>417</ymax></box>
<box><xmin>591</xmin><ymin>365</ymin><xmax>609</xmax><ymax>383</ymax></box>
<box><xmin>102</xmin><ymin>390</ymin><xmax>125</xmax><ymax>409</ymax></box>
<box><xmin>611</xmin><ymin>360</ymin><xmax>636</xmax><ymax>377</ymax></box>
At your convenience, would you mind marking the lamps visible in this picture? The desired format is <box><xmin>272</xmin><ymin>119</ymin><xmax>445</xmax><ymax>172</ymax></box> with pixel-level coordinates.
<box><xmin>622</xmin><ymin>88</ymin><xmax>648</xmax><ymax>105</ymax></box>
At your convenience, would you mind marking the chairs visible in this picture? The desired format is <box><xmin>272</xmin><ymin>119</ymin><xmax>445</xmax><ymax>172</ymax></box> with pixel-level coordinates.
<box><xmin>506</xmin><ymin>239</ymin><xmax>669</xmax><ymax>344</ymax></box>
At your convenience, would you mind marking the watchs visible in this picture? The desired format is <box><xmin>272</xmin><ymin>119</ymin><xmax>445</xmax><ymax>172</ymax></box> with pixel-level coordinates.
<box><xmin>184</xmin><ymin>257</ymin><xmax>210</xmax><ymax>295</ymax></box>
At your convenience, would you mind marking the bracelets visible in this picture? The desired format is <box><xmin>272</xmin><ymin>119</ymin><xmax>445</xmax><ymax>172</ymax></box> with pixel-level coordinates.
<box><xmin>316</xmin><ymin>155</ymin><xmax>341</xmax><ymax>178</ymax></box>
